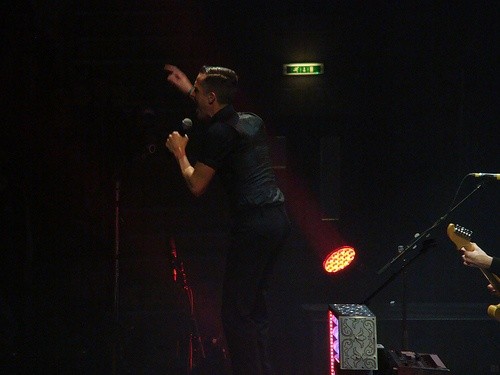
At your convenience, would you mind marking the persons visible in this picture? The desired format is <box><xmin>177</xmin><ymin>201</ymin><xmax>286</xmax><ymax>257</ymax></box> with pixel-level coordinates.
<box><xmin>164</xmin><ymin>64</ymin><xmax>290</xmax><ymax>375</ymax></box>
<box><xmin>459</xmin><ymin>241</ymin><xmax>500</xmax><ymax>297</ymax></box>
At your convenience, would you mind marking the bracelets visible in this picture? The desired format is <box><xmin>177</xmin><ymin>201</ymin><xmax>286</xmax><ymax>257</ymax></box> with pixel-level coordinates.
<box><xmin>185</xmin><ymin>85</ymin><xmax>193</xmax><ymax>95</ymax></box>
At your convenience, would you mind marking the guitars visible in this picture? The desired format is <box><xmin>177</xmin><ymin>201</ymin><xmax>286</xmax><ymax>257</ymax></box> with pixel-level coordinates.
<box><xmin>447</xmin><ymin>223</ymin><xmax>500</xmax><ymax>320</ymax></box>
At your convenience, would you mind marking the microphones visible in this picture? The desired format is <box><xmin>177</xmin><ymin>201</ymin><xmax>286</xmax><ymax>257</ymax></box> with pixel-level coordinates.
<box><xmin>168</xmin><ymin>118</ymin><xmax>192</xmax><ymax>161</ymax></box>
<box><xmin>470</xmin><ymin>173</ymin><xmax>500</xmax><ymax>180</ymax></box>
<box><xmin>166</xmin><ymin>237</ymin><xmax>177</xmax><ymax>281</ymax></box>
